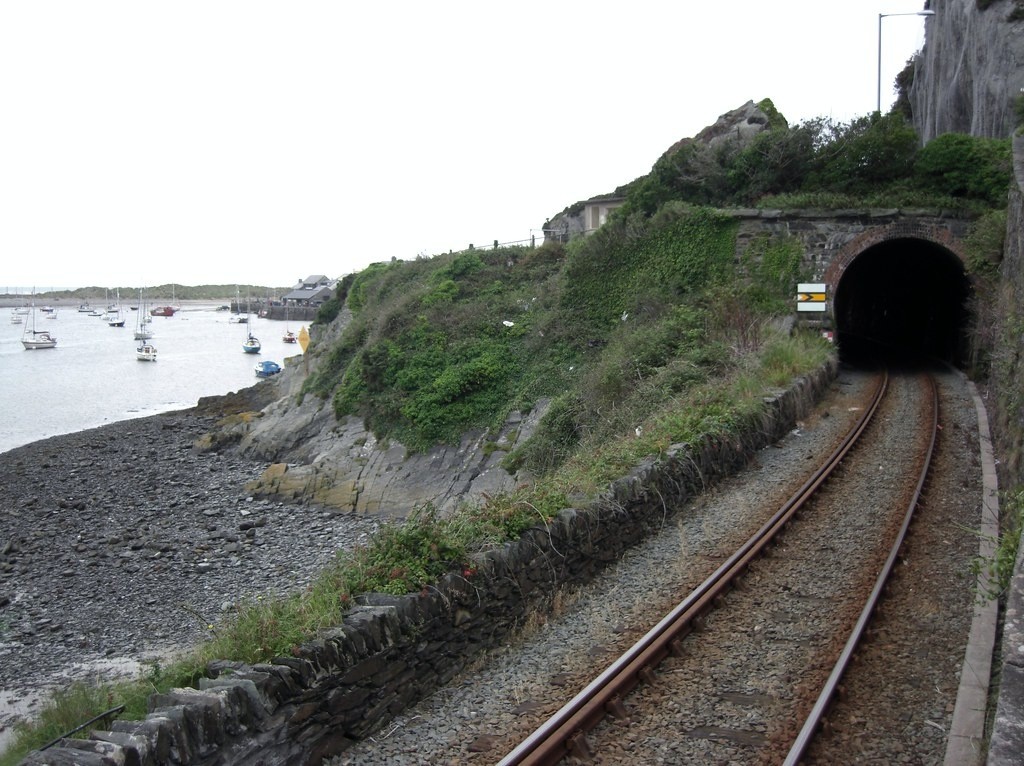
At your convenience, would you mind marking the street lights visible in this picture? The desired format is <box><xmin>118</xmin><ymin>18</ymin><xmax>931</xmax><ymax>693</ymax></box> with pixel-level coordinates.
<box><xmin>877</xmin><ymin>10</ymin><xmax>937</xmax><ymax>114</ymax></box>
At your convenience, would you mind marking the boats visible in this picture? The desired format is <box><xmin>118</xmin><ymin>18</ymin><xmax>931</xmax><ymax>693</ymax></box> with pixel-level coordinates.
<box><xmin>10</xmin><ymin>305</ymin><xmax>57</xmax><ymax>323</ymax></box>
<box><xmin>78</xmin><ymin>305</ymin><xmax>103</xmax><ymax>317</ymax></box>
<box><xmin>130</xmin><ymin>305</ymin><xmax>175</xmax><ymax>317</ymax></box>
<box><xmin>255</xmin><ymin>362</ymin><xmax>282</xmax><ymax>378</ymax></box>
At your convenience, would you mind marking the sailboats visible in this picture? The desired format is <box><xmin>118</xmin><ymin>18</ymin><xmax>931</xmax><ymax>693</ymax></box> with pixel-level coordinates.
<box><xmin>19</xmin><ymin>285</ymin><xmax>59</xmax><ymax>349</ymax></box>
<box><xmin>168</xmin><ymin>283</ymin><xmax>180</xmax><ymax>312</ymax></box>
<box><xmin>242</xmin><ymin>289</ymin><xmax>262</xmax><ymax>354</ymax></box>
<box><xmin>284</xmin><ymin>298</ymin><xmax>297</xmax><ymax>343</ymax></box>
<box><xmin>101</xmin><ymin>288</ymin><xmax>127</xmax><ymax>328</ymax></box>
<box><xmin>229</xmin><ymin>282</ymin><xmax>249</xmax><ymax>324</ymax></box>
<box><xmin>135</xmin><ymin>286</ymin><xmax>155</xmax><ymax>362</ymax></box>
